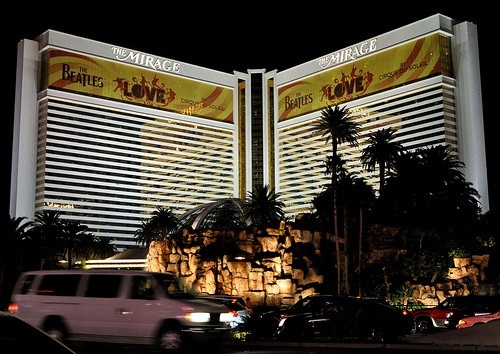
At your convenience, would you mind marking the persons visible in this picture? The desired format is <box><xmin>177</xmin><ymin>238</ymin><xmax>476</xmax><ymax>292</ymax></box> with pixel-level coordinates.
<box><xmin>297</xmin><ymin>295</ymin><xmax>303</xmax><ymax>307</ymax></box>
<box><xmin>245</xmin><ymin>297</ymin><xmax>253</xmax><ymax>309</ymax></box>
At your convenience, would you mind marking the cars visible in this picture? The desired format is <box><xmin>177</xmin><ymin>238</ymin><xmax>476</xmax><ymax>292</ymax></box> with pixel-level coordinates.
<box><xmin>199</xmin><ymin>294</ymin><xmax>500</xmax><ymax>344</ymax></box>
<box><xmin>8</xmin><ymin>269</ymin><xmax>250</xmax><ymax>354</ymax></box>
